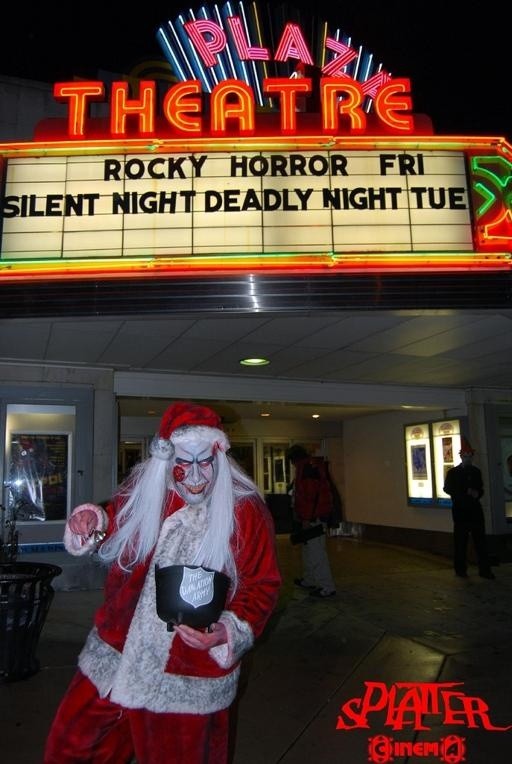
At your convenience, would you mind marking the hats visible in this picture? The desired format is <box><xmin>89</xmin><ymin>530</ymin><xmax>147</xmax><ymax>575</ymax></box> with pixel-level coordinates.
<box><xmin>150</xmin><ymin>401</ymin><xmax>231</xmax><ymax>459</ymax></box>
<box><xmin>458</xmin><ymin>434</ymin><xmax>474</xmax><ymax>457</ymax></box>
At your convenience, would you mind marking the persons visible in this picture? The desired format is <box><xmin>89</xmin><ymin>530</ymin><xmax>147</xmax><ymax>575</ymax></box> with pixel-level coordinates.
<box><xmin>287</xmin><ymin>445</ymin><xmax>337</xmax><ymax>596</ymax></box>
<box><xmin>443</xmin><ymin>447</ymin><xmax>496</xmax><ymax>580</ymax></box>
<box><xmin>43</xmin><ymin>400</ymin><xmax>283</xmax><ymax>764</ymax></box>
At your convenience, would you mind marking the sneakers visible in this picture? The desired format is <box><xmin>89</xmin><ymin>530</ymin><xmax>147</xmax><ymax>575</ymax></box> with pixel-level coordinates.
<box><xmin>309</xmin><ymin>588</ymin><xmax>336</xmax><ymax>598</ymax></box>
<box><xmin>294</xmin><ymin>577</ymin><xmax>316</xmax><ymax>588</ymax></box>
